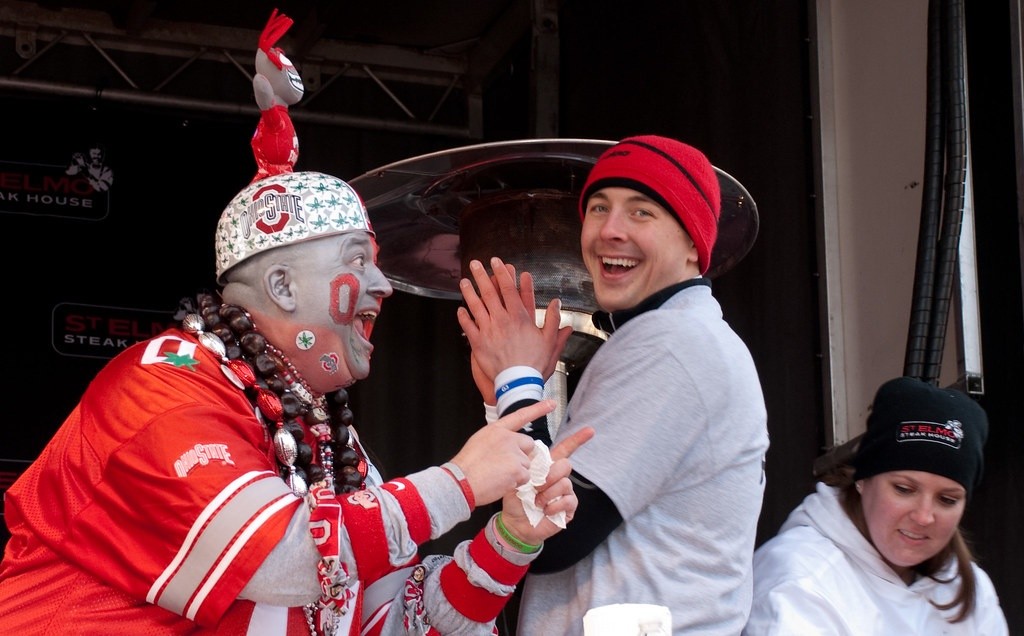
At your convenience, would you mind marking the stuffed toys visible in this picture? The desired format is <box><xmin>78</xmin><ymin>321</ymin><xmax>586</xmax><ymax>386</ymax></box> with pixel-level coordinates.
<box><xmin>248</xmin><ymin>8</ymin><xmax>304</xmax><ymax>180</ymax></box>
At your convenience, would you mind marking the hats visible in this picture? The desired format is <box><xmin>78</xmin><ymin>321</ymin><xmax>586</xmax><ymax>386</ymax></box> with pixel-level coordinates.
<box><xmin>852</xmin><ymin>376</ymin><xmax>988</xmax><ymax>502</ymax></box>
<box><xmin>214</xmin><ymin>8</ymin><xmax>376</xmax><ymax>285</ymax></box>
<box><xmin>578</xmin><ymin>135</ymin><xmax>720</xmax><ymax>277</ymax></box>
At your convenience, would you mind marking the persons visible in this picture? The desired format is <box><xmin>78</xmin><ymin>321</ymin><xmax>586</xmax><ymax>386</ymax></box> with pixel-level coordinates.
<box><xmin>0</xmin><ymin>172</ymin><xmax>595</xmax><ymax>636</ymax></box>
<box><xmin>748</xmin><ymin>377</ymin><xmax>1011</xmax><ymax>636</ymax></box>
<box><xmin>458</xmin><ymin>134</ymin><xmax>768</xmax><ymax>636</ymax></box>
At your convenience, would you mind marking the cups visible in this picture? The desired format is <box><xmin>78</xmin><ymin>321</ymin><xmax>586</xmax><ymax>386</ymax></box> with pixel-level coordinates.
<box><xmin>583</xmin><ymin>603</ymin><xmax>672</xmax><ymax>636</ymax></box>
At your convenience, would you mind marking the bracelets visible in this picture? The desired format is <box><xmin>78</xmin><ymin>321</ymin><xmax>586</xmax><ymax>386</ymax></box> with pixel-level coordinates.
<box><xmin>441</xmin><ymin>462</ymin><xmax>476</xmax><ymax>512</ymax></box>
<box><xmin>497</xmin><ymin>513</ymin><xmax>541</xmax><ymax>553</ymax></box>
<box><xmin>484</xmin><ymin>403</ymin><xmax>497</xmax><ymax>423</ymax></box>
<box><xmin>494</xmin><ymin>367</ymin><xmax>545</xmax><ymax>415</ymax></box>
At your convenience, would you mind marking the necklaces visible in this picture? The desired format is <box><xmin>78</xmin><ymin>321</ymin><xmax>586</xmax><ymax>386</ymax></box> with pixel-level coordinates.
<box><xmin>167</xmin><ymin>296</ymin><xmax>363</xmax><ymax>636</ymax></box>
